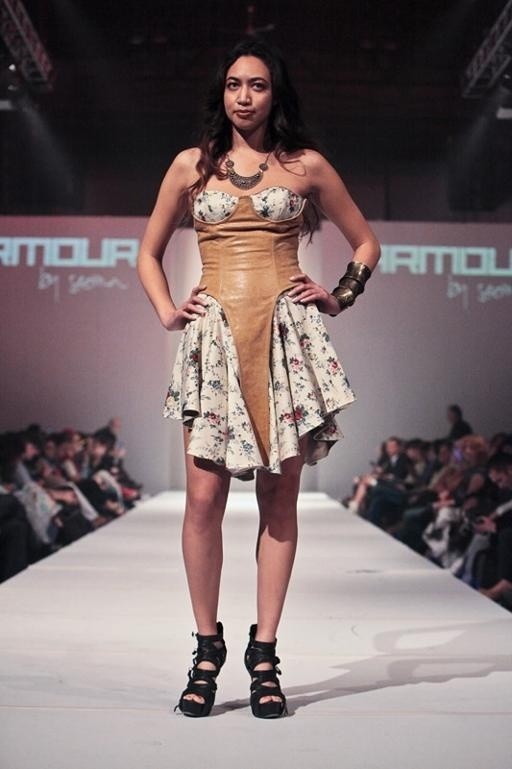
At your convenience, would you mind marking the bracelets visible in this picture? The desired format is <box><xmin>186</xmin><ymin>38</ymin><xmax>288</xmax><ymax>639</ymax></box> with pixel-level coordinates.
<box><xmin>330</xmin><ymin>261</ymin><xmax>371</xmax><ymax>312</ymax></box>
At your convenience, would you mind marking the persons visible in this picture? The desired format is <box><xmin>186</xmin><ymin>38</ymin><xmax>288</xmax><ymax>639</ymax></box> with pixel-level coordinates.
<box><xmin>136</xmin><ymin>35</ymin><xmax>384</xmax><ymax>719</ymax></box>
<box><xmin>0</xmin><ymin>416</ymin><xmax>147</xmax><ymax>587</ymax></box>
<box><xmin>342</xmin><ymin>405</ymin><xmax>512</xmax><ymax>613</ymax></box>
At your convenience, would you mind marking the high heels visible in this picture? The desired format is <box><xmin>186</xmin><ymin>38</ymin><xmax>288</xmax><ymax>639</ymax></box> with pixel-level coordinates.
<box><xmin>178</xmin><ymin>620</ymin><xmax>229</xmax><ymax>718</ymax></box>
<box><xmin>243</xmin><ymin>623</ymin><xmax>285</xmax><ymax>720</ymax></box>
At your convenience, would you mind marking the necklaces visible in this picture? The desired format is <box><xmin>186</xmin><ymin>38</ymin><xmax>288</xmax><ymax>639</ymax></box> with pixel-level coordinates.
<box><xmin>223</xmin><ymin>150</ymin><xmax>272</xmax><ymax>191</ymax></box>
